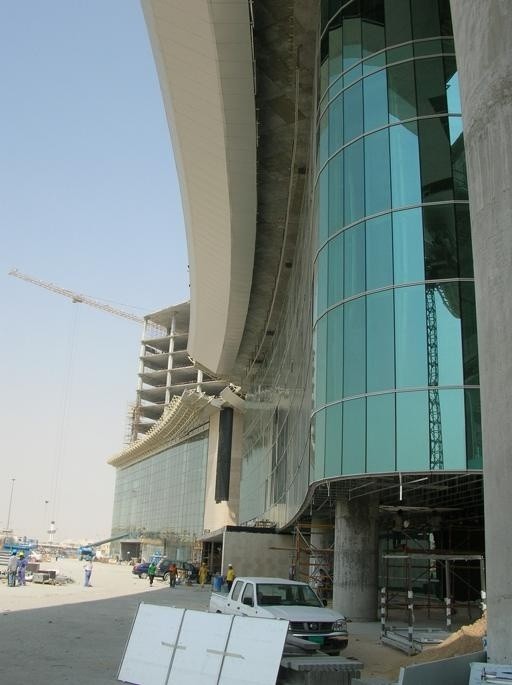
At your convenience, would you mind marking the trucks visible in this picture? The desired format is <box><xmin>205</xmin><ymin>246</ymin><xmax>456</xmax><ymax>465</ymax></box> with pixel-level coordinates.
<box><xmin>77</xmin><ymin>545</ymin><xmax>93</xmax><ymax>561</ymax></box>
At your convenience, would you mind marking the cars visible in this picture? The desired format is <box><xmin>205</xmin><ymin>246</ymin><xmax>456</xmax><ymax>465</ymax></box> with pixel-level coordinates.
<box><xmin>132</xmin><ymin>562</ymin><xmax>153</xmax><ymax>579</ymax></box>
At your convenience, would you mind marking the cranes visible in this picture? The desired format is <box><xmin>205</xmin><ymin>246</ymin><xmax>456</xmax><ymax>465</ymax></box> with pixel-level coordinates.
<box><xmin>9</xmin><ymin>269</ymin><xmax>175</xmax><ymax>335</ymax></box>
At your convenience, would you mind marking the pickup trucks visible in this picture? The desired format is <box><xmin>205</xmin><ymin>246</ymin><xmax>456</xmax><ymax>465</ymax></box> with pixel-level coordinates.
<box><xmin>209</xmin><ymin>576</ymin><xmax>348</xmax><ymax>659</ymax></box>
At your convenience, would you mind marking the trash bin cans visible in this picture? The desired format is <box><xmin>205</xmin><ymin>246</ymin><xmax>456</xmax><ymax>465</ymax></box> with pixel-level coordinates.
<box><xmin>213</xmin><ymin>575</ymin><xmax>222</xmax><ymax>592</ymax></box>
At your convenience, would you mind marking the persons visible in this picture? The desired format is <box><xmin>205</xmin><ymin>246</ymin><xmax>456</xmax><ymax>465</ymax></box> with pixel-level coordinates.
<box><xmin>6</xmin><ymin>549</ymin><xmax>19</xmax><ymax>587</ymax></box>
<box><xmin>147</xmin><ymin>561</ymin><xmax>158</xmax><ymax>587</ymax></box>
<box><xmin>198</xmin><ymin>562</ymin><xmax>209</xmax><ymax>589</ymax></box>
<box><xmin>17</xmin><ymin>551</ymin><xmax>28</xmax><ymax>586</ymax></box>
<box><xmin>82</xmin><ymin>557</ymin><xmax>93</xmax><ymax>587</ymax></box>
<box><xmin>169</xmin><ymin>563</ymin><xmax>179</xmax><ymax>588</ymax></box>
<box><xmin>315</xmin><ymin>569</ymin><xmax>328</xmax><ymax>606</ymax></box>
<box><xmin>226</xmin><ymin>563</ymin><xmax>236</xmax><ymax>593</ymax></box>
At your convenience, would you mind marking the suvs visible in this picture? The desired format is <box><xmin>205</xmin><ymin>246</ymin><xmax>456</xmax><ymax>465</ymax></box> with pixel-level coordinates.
<box><xmin>151</xmin><ymin>556</ymin><xmax>197</xmax><ymax>582</ymax></box>
